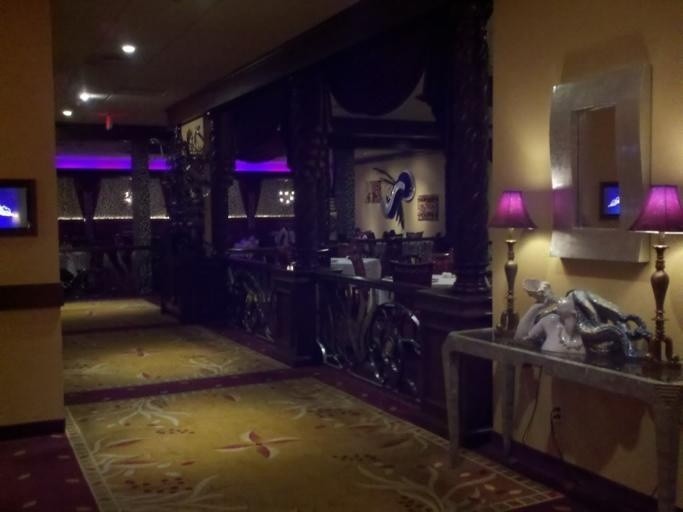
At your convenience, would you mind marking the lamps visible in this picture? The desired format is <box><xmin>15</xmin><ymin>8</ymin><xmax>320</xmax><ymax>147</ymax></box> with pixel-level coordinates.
<box><xmin>628</xmin><ymin>183</ymin><xmax>682</xmax><ymax>371</ymax></box>
<box><xmin>487</xmin><ymin>190</ymin><xmax>538</xmax><ymax>332</ymax></box>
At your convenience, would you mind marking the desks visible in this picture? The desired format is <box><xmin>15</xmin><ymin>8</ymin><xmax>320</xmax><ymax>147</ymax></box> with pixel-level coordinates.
<box><xmin>439</xmin><ymin>320</ymin><xmax>683</xmax><ymax>506</ymax></box>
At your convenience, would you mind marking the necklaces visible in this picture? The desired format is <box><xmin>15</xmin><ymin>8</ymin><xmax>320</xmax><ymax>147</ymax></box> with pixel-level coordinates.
<box><xmin>558</xmin><ymin>328</ymin><xmax>583</xmax><ymax>350</ymax></box>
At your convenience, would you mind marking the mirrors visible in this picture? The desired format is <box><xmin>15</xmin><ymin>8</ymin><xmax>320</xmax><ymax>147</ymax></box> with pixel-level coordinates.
<box><xmin>544</xmin><ymin>60</ymin><xmax>656</xmax><ymax>270</ymax></box>
<box><xmin>209</xmin><ymin>100</ymin><xmax>314</xmax><ymax>254</ymax></box>
<box><xmin>321</xmin><ymin>60</ymin><xmax>456</xmax><ymax>270</ymax></box>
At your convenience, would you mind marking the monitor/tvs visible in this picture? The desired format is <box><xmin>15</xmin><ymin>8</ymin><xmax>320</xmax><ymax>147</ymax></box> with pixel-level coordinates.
<box><xmin>599</xmin><ymin>182</ymin><xmax>620</xmax><ymax>216</ymax></box>
<box><xmin>0</xmin><ymin>179</ymin><xmax>38</xmax><ymax>236</ymax></box>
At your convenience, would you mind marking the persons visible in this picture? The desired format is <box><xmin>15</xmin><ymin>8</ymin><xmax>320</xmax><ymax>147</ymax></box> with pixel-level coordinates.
<box><xmin>514</xmin><ymin>278</ymin><xmax>653</xmax><ymax>363</ymax></box>
